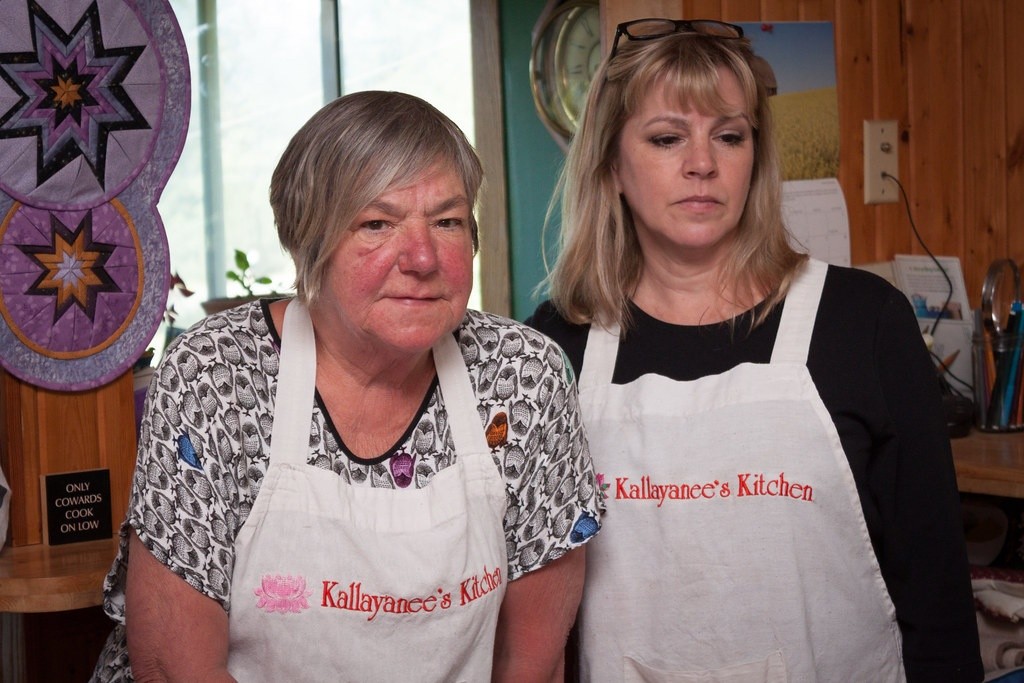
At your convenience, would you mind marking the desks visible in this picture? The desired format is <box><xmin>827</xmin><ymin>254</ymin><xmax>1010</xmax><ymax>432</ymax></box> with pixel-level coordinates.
<box><xmin>950</xmin><ymin>426</ymin><xmax>1024</xmax><ymax>683</ymax></box>
<box><xmin>0</xmin><ymin>536</ymin><xmax>129</xmax><ymax>683</ymax></box>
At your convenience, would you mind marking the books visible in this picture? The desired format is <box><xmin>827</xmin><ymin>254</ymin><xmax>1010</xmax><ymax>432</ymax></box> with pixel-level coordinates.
<box><xmin>856</xmin><ymin>254</ymin><xmax>977</xmax><ymax>401</ymax></box>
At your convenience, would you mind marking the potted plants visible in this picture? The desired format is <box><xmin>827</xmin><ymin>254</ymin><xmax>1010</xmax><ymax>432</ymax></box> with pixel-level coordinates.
<box><xmin>199</xmin><ymin>251</ymin><xmax>293</xmax><ymax>315</ymax></box>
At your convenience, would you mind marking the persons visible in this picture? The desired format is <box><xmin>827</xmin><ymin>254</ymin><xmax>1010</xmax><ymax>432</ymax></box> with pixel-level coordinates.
<box><xmin>523</xmin><ymin>16</ymin><xmax>986</xmax><ymax>683</ymax></box>
<box><xmin>88</xmin><ymin>93</ymin><xmax>607</xmax><ymax>683</ymax></box>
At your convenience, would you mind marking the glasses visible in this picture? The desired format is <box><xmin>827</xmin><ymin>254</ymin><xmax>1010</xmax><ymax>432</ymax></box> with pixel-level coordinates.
<box><xmin>607</xmin><ymin>17</ymin><xmax>744</xmax><ymax>62</ymax></box>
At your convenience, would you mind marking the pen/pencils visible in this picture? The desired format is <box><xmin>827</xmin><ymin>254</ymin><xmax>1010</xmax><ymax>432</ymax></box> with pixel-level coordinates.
<box><xmin>922</xmin><ymin>325</ymin><xmax>930</xmax><ymax>335</ymax></box>
<box><xmin>974</xmin><ymin>299</ymin><xmax>1024</xmax><ymax>430</ymax></box>
<box><xmin>936</xmin><ymin>350</ymin><xmax>960</xmax><ymax>371</ymax></box>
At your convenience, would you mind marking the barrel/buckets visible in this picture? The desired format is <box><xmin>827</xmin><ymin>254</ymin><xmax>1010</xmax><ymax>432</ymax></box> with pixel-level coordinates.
<box><xmin>970</xmin><ymin>258</ymin><xmax>1024</xmax><ymax>435</ymax></box>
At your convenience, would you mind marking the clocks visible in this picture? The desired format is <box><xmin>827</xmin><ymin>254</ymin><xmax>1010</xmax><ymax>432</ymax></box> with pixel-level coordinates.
<box><xmin>526</xmin><ymin>0</ymin><xmax>602</xmax><ymax>149</ymax></box>
